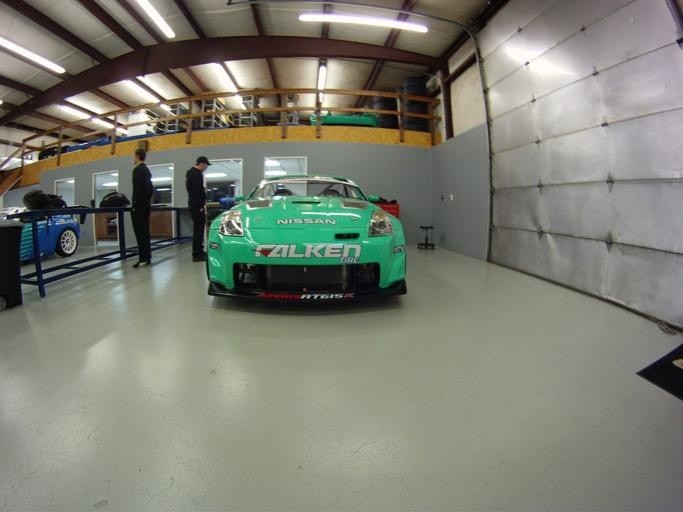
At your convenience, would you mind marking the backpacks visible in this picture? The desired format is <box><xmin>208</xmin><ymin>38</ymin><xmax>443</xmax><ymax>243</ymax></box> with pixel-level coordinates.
<box><xmin>23</xmin><ymin>190</ymin><xmax>67</xmax><ymax>209</ymax></box>
<box><xmin>100</xmin><ymin>191</ymin><xmax>130</xmax><ymax>208</ymax></box>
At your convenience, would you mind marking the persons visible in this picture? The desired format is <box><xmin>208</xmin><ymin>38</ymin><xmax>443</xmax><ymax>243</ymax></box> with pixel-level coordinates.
<box><xmin>184</xmin><ymin>156</ymin><xmax>212</xmax><ymax>262</ymax></box>
<box><xmin>130</xmin><ymin>149</ymin><xmax>153</xmax><ymax>268</ymax></box>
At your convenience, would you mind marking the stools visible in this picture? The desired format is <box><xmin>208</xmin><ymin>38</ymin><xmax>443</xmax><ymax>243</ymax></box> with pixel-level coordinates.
<box><xmin>417</xmin><ymin>225</ymin><xmax>436</xmax><ymax>249</ymax></box>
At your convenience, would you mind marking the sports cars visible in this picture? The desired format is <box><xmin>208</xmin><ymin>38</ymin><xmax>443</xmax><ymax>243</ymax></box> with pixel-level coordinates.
<box><xmin>203</xmin><ymin>174</ymin><xmax>409</xmax><ymax>306</ymax></box>
<box><xmin>0</xmin><ymin>202</ymin><xmax>90</xmax><ymax>263</ymax></box>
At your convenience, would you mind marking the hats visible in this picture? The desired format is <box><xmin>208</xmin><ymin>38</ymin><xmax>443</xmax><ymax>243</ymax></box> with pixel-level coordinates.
<box><xmin>197</xmin><ymin>156</ymin><xmax>211</xmax><ymax>165</ymax></box>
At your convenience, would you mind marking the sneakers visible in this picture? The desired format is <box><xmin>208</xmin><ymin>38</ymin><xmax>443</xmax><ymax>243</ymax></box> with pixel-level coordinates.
<box><xmin>133</xmin><ymin>260</ymin><xmax>152</xmax><ymax>267</ymax></box>
<box><xmin>193</xmin><ymin>253</ymin><xmax>206</xmax><ymax>261</ymax></box>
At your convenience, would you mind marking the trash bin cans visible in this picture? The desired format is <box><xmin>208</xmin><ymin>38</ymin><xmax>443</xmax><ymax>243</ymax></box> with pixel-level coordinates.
<box><xmin>0</xmin><ymin>218</ymin><xmax>24</xmax><ymax>311</ymax></box>
